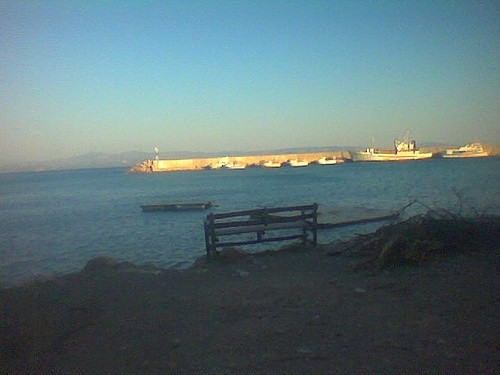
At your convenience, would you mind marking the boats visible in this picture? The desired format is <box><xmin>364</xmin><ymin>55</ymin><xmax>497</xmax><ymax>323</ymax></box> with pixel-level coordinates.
<box><xmin>442</xmin><ymin>143</ymin><xmax>489</xmax><ymax>158</ymax></box>
<box><xmin>356</xmin><ymin>137</ymin><xmax>434</xmax><ymax>161</ymax></box>
<box><xmin>131</xmin><ymin>147</ymin><xmax>351</xmax><ymax>173</ymax></box>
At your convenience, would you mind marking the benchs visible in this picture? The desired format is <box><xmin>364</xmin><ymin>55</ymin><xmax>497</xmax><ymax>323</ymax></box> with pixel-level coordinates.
<box><xmin>204</xmin><ymin>203</ymin><xmax>318</xmax><ymax>262</ymax></box>
